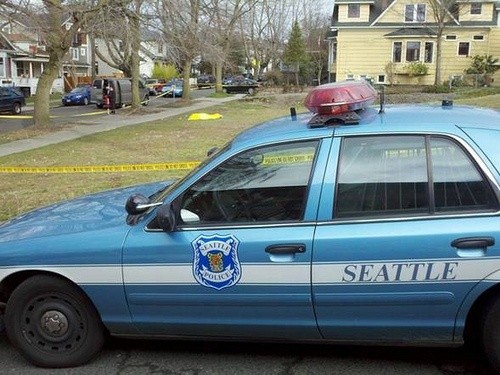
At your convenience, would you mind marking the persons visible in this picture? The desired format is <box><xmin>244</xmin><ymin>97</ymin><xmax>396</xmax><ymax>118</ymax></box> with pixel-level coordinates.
<box><xmin>103</xmin><ymin>86</ymin><xmax>115</xmax><ymax>113</ymax></box>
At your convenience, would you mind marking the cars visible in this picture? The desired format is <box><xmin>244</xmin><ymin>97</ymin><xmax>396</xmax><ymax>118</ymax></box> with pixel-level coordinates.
<box><xmin>0</xmin><ymin>80</ymin><xmax>499</xmax><ymax>374</ymax></box>
<box><xmin>143</xmin><ymin>77</ymin><xmax>169</xmax><ymax>92</ymax></box>
<box><xmin>0</xmin><ymin>86</ymin><xmax>27</xmax><ymax>115</ymax></box>
<box><xmin>162</xmin><ymin>79</ymin><xmax>185</xmax><ymax>97</ymax></box>
<box><xmin>61</xmin><ymin>86</ymin><xmax>91</xmax><ymax>106</ymax></box>
<box><xmin>198</xmin><ymin>72</ymin><xmax>261</xmax><ymax>94</ymax></box>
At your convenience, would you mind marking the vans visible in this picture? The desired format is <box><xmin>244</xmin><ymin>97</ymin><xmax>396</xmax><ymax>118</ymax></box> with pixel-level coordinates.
<box><xmin>89</xmin><ymin>77</ymin><xmax>150</xmax><ymax>108</ymax></box>
